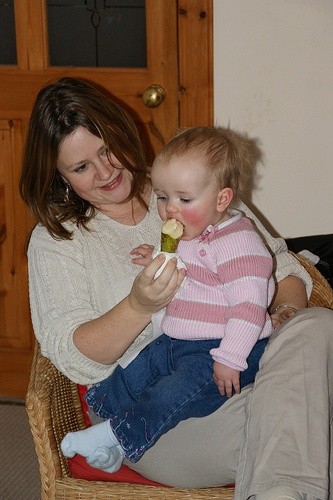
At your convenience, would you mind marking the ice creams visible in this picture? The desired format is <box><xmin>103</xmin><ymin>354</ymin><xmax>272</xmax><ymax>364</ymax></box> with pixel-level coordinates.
<box><xmin>160</xmin><ymin>218</ymin><xmax>184</xmax><ymax>254</ymax></box>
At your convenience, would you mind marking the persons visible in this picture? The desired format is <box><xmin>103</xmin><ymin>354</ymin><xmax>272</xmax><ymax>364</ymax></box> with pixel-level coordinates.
<box><xmin>18</xmin><ymin>75</ymin><xmax>331</xmax><ymax>500</ymax></box>
<box><xmin>59</xmin><ymin>122</ymin><xmax>274</xmax><ymax>474</ymax></box>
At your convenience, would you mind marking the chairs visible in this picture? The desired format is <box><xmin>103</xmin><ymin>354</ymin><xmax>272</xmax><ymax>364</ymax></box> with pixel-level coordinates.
<box><xmin>24</xmin><ymin>248</ymin><xmax>333</xmax><ymax>500</ymax></box>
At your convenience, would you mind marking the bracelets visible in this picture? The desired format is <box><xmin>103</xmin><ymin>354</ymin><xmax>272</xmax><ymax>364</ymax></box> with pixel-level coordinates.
<box><xmin>272</xmin><ymin>304</ymin><xmax>298</xmax><ymax>314</ymax></box>
